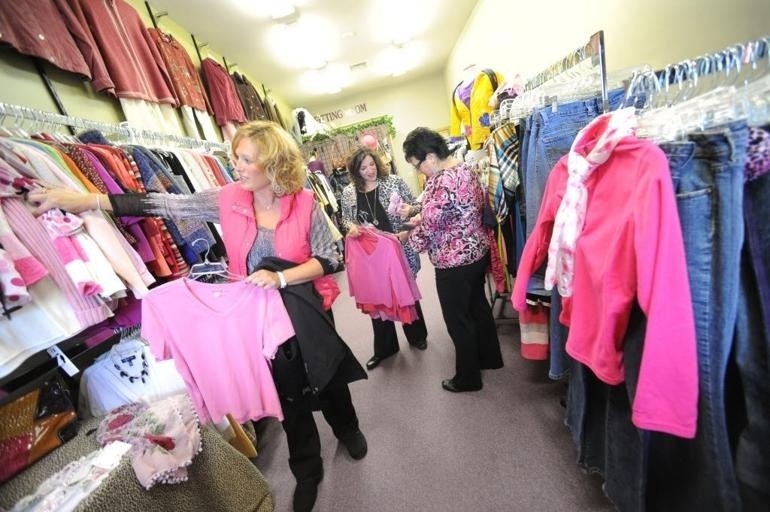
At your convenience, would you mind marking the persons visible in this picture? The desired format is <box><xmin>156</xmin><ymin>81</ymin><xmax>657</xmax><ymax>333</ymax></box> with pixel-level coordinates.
<box><xmin>398</xmin><ymin>127</ymin><xmax>503</xmax><ymax>393</ymax></box>
<box><xmin>27</xmin><ymin>120</ymin><xmax>367</xmax><ymax>512</ymax></box>
<box><xmin>447</xmin><ymin>64</ymin><xmax>506</xmax><ymax>148</ymax></box>
<box><xmin>343</xmin><ymin>147</ymin><xmax>428</xmax><ymax>369</ymax></box>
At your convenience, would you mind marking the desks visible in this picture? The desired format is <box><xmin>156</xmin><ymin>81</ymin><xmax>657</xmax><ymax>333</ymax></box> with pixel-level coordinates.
<box><xmin>1</xmin><ymin>394</ymin><xmax>214</xmax><ymax>510</ymax></box>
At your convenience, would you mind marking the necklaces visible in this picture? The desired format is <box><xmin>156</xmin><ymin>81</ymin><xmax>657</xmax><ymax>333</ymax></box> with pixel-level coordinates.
<box><xmin>364</xmin><ymin>182</ymin><xmax>378</xmax><ymax>226</ymax></box>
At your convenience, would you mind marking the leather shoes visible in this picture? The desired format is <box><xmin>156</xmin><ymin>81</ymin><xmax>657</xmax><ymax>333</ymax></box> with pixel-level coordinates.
<box><xmin>293</xmin><ymin>478</ymin><xmax>317</xmax><ymax>511</ymax></box>
<box><xmin>342</xmin><ymin>428</ymin><xmax>368</xmax><ymax>460</ymax></box>
<box><xmin>366</xmin><ymin>354</ymin><xmax>384</xmax><ymax>370</ymax></box>
<box><xmin>442</xmin><ymin>374</ymin><xmax>483</xmax><ymax>392</ymax></box>
<box><xmin>413</xmin><ymin>336</ymin><xmax>427</xmax><ymax>350</ymax></box>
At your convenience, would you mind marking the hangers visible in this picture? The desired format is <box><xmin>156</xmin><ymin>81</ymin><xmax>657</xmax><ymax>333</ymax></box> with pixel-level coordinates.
<box><xmin>185</xmin><ymin>262</ymin><xmax>246</xmax><ymax>289</ymax></box>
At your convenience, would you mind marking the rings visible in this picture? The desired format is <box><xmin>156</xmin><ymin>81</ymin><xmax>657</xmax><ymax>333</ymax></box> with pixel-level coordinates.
<box><xmin>41</xmin><ymin>187</ymin><xmax>46</xmax><ymax>194</ymax></box>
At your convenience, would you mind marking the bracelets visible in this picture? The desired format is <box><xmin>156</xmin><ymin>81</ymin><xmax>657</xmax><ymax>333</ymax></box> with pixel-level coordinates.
<box><xmin>96</xmin><ymin>193</ymin><xmax>100</xmax><ymax>210</ymax></box>
<box><xmin>276</xmin><ymin>270</ymin><xmax>288</xmax><ymax>290</ymax></box>
